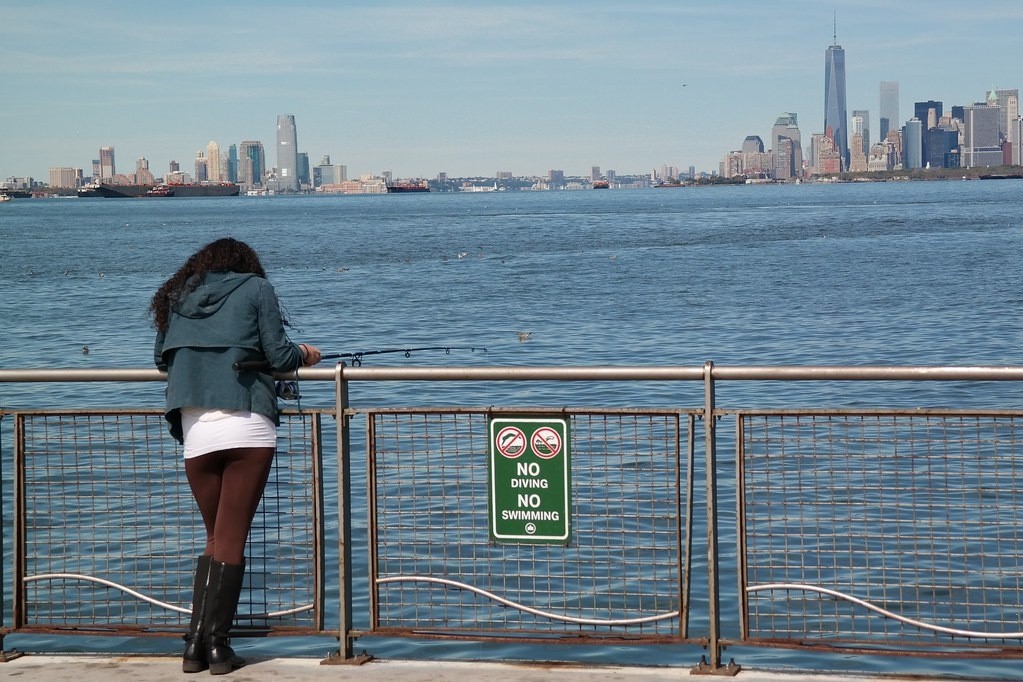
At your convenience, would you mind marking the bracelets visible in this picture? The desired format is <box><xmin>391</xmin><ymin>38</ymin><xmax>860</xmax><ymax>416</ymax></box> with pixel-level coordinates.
<box><xmin>299</xmin><ymin>344</ymin><xmax>309</xmax><ymax>360</ymax></box>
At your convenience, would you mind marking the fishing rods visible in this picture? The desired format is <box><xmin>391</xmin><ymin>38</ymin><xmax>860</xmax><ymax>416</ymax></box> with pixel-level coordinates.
<box><xmin>319</xmin><ymin>346</ymin><xmax>488</xmax><ymax>361</ymax></box>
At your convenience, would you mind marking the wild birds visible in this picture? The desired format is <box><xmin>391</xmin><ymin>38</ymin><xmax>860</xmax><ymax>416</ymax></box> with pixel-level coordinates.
<box><xmin>81</xmin><ymin>346</ymin><xmax>88</xmax><ymax>351</ymax></box>
<box><xmin>516</xmin><ymin>332</ymin><xmax>532</xmax><ymax>338</ymax></box>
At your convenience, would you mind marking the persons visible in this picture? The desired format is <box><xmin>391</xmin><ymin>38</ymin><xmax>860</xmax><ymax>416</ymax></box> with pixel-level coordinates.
<box><xmin>147</xmin><ymin>237</ymin><xmax>322</xmax><ymax>676</ymax></box>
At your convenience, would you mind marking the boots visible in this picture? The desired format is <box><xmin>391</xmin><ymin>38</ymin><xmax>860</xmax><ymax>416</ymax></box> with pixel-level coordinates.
<box><xmin>202</xmin><ymin>558</ymin><xmax>246</xmax><ymax>674</ymax></box>
<box><xmin>182</xmin><ymin>554</ymin><xmax>213</xmax><ymax>672</ymax></box>
